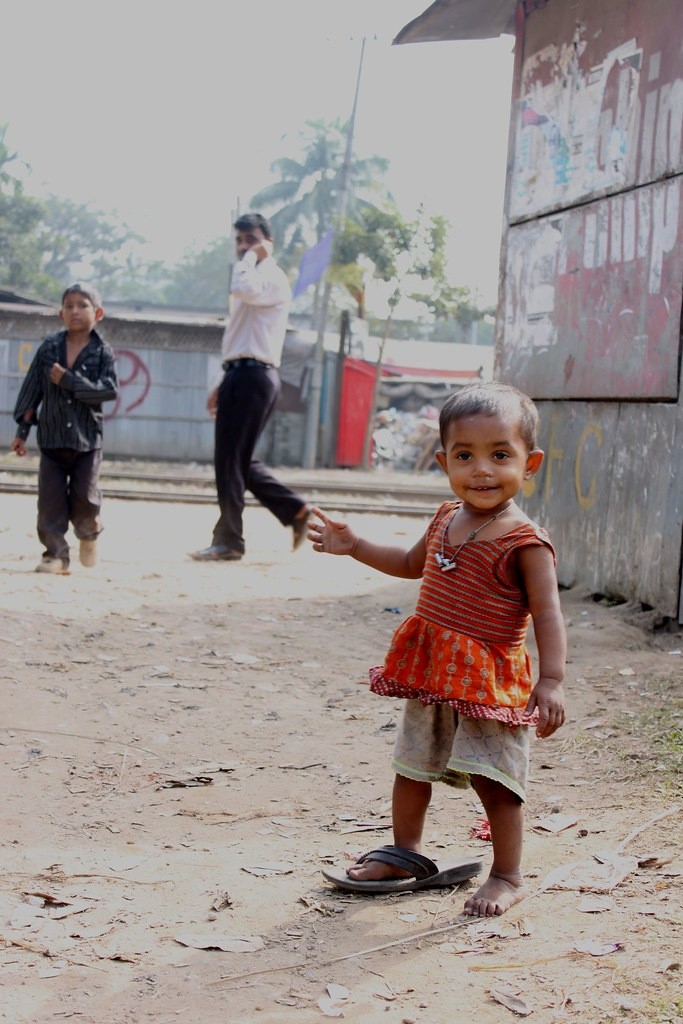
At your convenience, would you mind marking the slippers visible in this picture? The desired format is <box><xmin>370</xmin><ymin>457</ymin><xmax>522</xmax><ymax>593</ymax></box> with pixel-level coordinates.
<box><xmin>322</xmin><ymin>844</ymin><xmax>484</xmax><ymax>890</ymax></box>
<box><xmin>291</xmin><ymin>508</ymin><xmax>317</xmax><ymax>552</ymax></box>
<box><xmin>188</xmin><ymin>543</ymin><xmax>242</xmax><ymax>560</ymax></box>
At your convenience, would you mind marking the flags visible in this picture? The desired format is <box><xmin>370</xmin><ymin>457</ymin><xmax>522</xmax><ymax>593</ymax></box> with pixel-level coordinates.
<box><xmin>292</xmin><ymin>228</ymin><xmax>333</xmax><ymax>296</ymax></box>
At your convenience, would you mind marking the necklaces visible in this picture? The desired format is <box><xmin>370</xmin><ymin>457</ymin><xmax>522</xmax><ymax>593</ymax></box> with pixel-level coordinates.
<box><xmin>433</xmin><ymin>498</ymin><xmax>515</xmax><ymax>572</ymax></box>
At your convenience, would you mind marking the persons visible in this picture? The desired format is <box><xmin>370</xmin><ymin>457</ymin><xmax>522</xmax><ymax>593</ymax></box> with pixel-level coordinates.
<box><xmin>306</xmin><ymin>379</ymin><xmax>569</xmax><ymax>917</ymax></box>
<box><xmin>185</xmin><ymin>211</ymin><xmax>316</xmax><ymax>562</ymax></box>
<box><xmin>8</xmin><ymin>282</ymin><xmax>122</xmax><ymax>577</ymax></box>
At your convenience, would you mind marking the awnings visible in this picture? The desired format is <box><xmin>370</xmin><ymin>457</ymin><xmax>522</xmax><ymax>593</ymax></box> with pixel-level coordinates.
<box><xmin>390</xmin><ymin>0</ymin><xmax>518</xmax><ymax>46</ymax></box>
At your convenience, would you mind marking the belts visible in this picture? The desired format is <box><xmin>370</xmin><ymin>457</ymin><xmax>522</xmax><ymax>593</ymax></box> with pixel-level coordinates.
<box><xmin>222</xmin><ymin>358</ymin><xmax>273</xmax><ymax>370</ymax></box>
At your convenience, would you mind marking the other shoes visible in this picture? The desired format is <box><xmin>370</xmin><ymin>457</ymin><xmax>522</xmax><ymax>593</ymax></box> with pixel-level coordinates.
<box><xmin>35</xmin><ymin>557</ymin><xmax>71</xmax><ymax>576</ymax></box>
<box><xmin>79</xmin><ymin>538</ymin><xmax>96</xmax><ymax>568</ymax></box>
